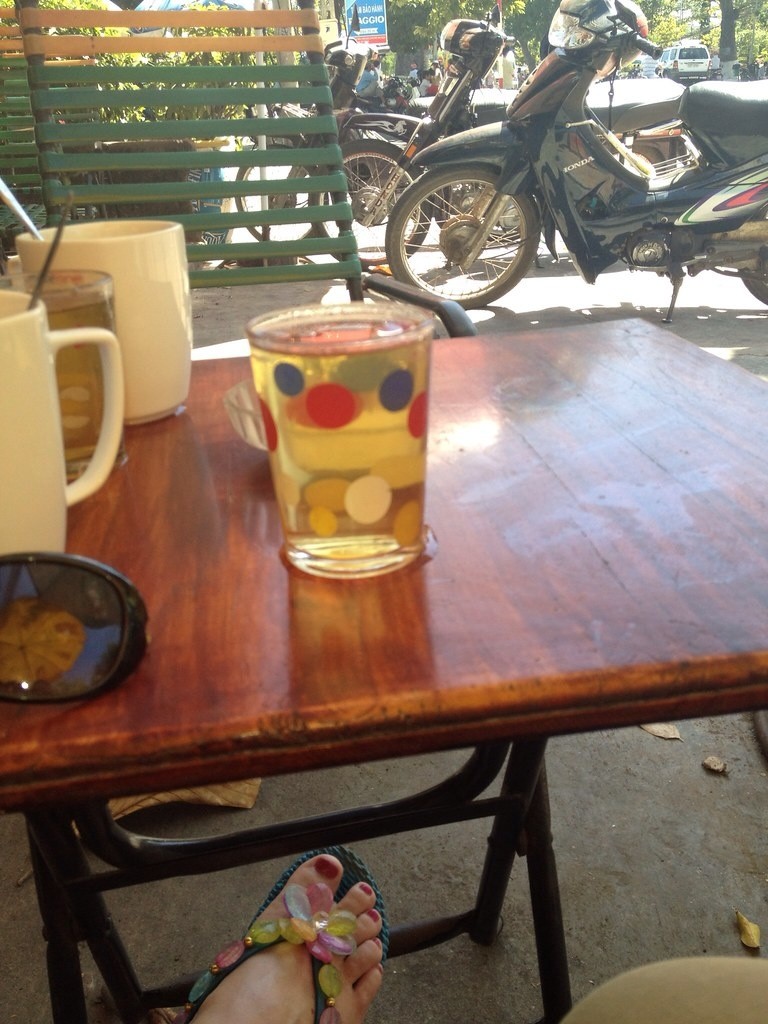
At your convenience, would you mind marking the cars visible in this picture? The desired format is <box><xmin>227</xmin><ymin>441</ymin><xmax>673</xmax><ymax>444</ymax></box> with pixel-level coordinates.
<box><xmin>634</xmin><ymin>55</ymin><xmax>658</xmax><ymax>78</ymax></box>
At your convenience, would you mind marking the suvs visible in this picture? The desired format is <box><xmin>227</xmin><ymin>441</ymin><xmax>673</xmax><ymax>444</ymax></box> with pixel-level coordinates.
<box><xmin>654</xmin><ymin>43</ymin><xmax>711</xmax><ymax>84</ymax></box>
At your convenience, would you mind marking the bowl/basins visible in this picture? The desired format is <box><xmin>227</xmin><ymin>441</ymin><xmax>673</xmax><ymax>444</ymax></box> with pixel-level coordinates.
<box><xmin>224</xmin><ymin>379</ymin><xmax>267</xmax><ymax>453</ymax></box>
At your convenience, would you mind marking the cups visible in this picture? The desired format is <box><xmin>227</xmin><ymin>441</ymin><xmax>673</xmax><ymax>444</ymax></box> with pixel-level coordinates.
<box><xmin>15</xmin><ymin>221</ymin><xmax>193</xmax><ymax>425</ymax></box>
<box><xmin>0</xmin><ymin>269</ymin><xmax>129</xmax><ymax>480</ymax></box>
<box><xmin>0</xmin><ymin>289</ymin><xmax>125</xmax><ymax>554</ymax></box>
<box><xmin>246</xmin><ymin>302</ymin><xmax>438</xmax><ymax>579</ymax></box>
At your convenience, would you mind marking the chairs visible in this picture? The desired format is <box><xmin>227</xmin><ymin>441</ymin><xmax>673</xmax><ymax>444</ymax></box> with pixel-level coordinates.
<box><xmin>0</xmin><ymin>1</ymin><xmax>479</xmax><ymax>351</ymax></box>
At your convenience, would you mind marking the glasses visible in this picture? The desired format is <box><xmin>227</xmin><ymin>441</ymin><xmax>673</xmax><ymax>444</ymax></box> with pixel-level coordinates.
<box><xmin>0</xmin><ymin>552</ymin><xmax>149</xmax><ymax>705</ymax></box>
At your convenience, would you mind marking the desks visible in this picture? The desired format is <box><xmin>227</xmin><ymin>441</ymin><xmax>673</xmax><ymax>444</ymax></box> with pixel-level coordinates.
<box><xmin>2</xmin><ymin>314</ymin><xmax>767</xmax><ymax>1024</ymax></box>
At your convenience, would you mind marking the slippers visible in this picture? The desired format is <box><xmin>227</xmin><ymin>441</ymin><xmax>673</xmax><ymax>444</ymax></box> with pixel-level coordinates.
<box><xmin>182</xmin><ymin>844</ymin><xmax>389</xmax><ymax>1024</ymax></box>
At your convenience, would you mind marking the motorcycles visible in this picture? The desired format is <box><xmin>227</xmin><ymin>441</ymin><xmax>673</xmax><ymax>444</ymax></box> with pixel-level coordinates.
<box><xmin>384</xmin><ymin>0</ymin><xmax>768</xmax><ymax>323</ymax></box>
<box><xmin>232</xmin><ymin>38</ymin><xmax>523</xmax><ymax>244</ymax></box>
<box><xmin>310</xmin><ymin>5</ymin><xmax>686</xmax><ymax>270</ymax></box>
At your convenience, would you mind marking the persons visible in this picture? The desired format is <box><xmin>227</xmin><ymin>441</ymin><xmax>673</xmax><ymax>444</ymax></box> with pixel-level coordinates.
<box><xmin>711</xmin><ymin>51</ymin><xmax>768</xmax><ymax>80</ymax></box>
<box><xmin>170</xmin><ymin>847</ymin><xmax>388</xmax><ymax>1024</ymax></box>
<box><xmin>484</xmin><ymin>46</ymin><xmax>515</xmax><ymax>89</ymax></box>
<box><xmin>356</xmin><ymin>59</ymin><xmax>444</xmax><ymax>98</ymax></box>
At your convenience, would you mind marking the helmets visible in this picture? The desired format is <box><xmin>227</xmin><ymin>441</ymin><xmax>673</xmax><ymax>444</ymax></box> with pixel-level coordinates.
<box><xmin>611</xmin><ymin>0</ymin><xmax>648</xmax><ymax>68</ymax></box>
